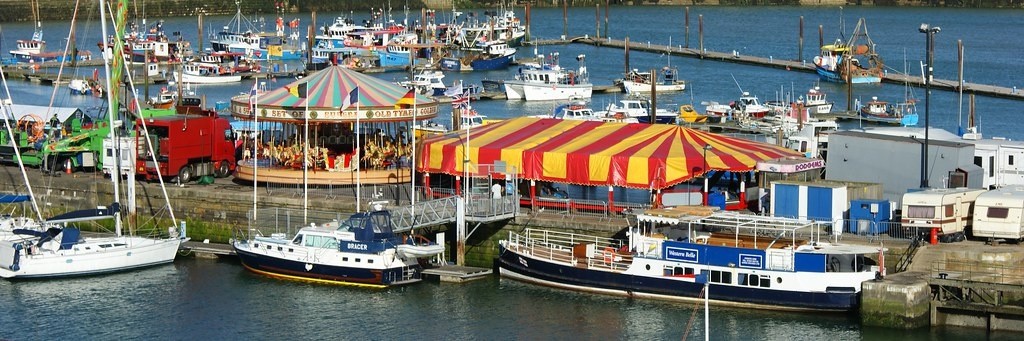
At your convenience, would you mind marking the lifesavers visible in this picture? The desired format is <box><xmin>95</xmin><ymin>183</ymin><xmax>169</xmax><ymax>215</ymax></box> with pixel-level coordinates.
<box><xmin>92</xmin><ymin>86</ymin><xmax>102</xmax><ymax>98</ymax></box>
<box><xmin>604</xmin><ymin>248</ymin><xmax>614</xmax><ymax>262</ymax></box>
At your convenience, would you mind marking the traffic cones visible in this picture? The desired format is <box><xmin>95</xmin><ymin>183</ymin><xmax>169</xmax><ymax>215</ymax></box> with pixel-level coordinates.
<box><xmin>66</xmin><ymin>158</ymin><xmax>73</xmax><ymax>175</ymax></box>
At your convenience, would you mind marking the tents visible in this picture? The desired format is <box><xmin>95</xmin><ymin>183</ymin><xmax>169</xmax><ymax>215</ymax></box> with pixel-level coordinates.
<box><xmin>415</xmin><ymin>116</ymin><xmax>809</xmax><ymax>214</ymax></box>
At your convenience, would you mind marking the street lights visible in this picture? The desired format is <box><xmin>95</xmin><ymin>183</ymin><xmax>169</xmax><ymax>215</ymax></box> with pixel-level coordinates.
<box><xmin>918</xmin><ymin>22</ymin><xmax>942</xmax><ymax>187</ymax></box>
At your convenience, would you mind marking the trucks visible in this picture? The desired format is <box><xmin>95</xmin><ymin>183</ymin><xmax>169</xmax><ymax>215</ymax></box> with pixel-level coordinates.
<box><xmin>134</xmin><ymin>103</ymin><xmax>237</xmax><ymax>186</ymax></box>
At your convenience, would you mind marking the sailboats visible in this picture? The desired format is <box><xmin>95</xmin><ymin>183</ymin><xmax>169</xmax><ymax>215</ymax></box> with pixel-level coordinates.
<box><xmin>0</xmin><ymin>0</ymin><xmax>922</xmax><ymax>313</ymax></box>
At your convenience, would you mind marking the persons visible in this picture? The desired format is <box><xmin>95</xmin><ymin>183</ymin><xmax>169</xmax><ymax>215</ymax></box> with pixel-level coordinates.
<box><xmin>489</xmin><ymin>179</ymin><xmax>559</xmax><ymax>200</ymax></box>
<box><xmin>49</xmin><ymin>114</ymin><xmax>60</xmax><ymax>128</ymax></box>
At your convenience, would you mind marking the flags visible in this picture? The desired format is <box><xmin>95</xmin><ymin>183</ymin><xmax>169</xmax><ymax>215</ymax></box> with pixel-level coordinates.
<box><xmin>443</xmin><ymin>84</ymin><xmax>463</xmax><ymax>97</ymax></box>
<box><xmin>452</xmin><ymin>91</ymin><xmax>469</xmax><ymax>109</ymax></box>
<box><xmin>248</xmin><ymin>80</ymin><xmax>257</xmax><ymax>118</ymax></box>
<box><xmin>283</xmin><ymin>82</ymin><xmax>307</xmax><ymax>98</ymax></box>
<box><xmin>340</xmin><ymin>87</ymin><xmax>358</xmax><ymax>116</ymax></box>
<box><xmin>394</xmin><ymin>88</ymin><xmax>415</xmax><ymax>110</ymax></box>
